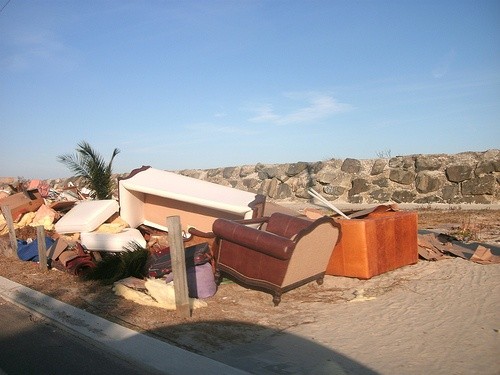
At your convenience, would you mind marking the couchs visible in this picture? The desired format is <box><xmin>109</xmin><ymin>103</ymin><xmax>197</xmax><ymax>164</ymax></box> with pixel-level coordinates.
<box><xmin>322</xmin><ymin>209</ymin><xmax>420</xmax><ymax>280</ymax></box>
<box><xmin>212</xmin><ymin>212</ymin><xmax>343</xmax><ymax>306</ymax></box>
<box><xmin>118</xmin><ymin>165</ymin><xmax>266</xmax><ymax>242</ymax></box>
